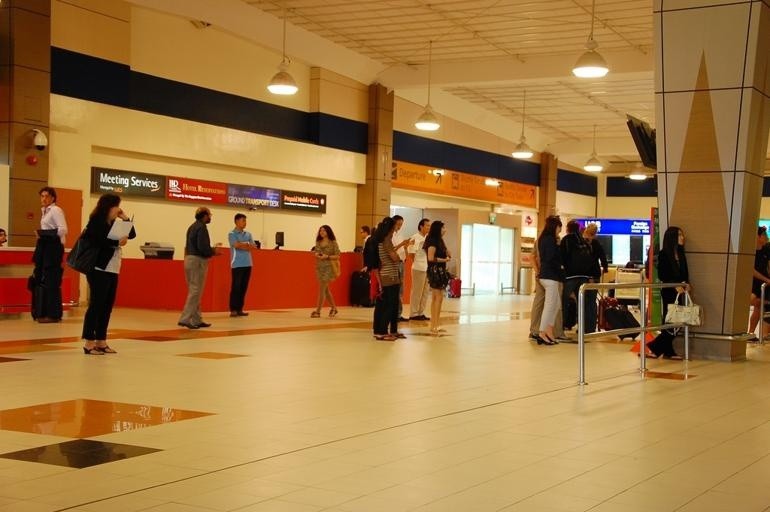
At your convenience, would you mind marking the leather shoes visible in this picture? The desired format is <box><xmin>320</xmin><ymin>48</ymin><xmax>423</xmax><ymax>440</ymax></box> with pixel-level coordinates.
<box><xmin>36</xmin><ymin>315</ymin><xmax>57</xmax><ymax>323</ymax></box>
<box><xmin>178</xmin><ymin>322</ymin><xmax>200</xmax><ymax>329</ymax></box>
<box><xmin>230</xmin><ymin>311</ymin><xmax>238</xmax><ymax>317</ymax></box>
<box><xmin>423</xmin><ymin>315</ymin><xmax>430</xmax><ymax>320</ymax></box>
<box><xmin>239</xmin><ymin>311</ymin><xmax>248</xmax><ymax>316</ymax></box>
<box><xmin>409</xmin><ymin>316</ymin><xmax>424</xmax><ymax>320</ymax></box>
<box><xmin>198</xmin><ymin>322</ymin><xmax>211</xmax><ymax>327</ymax></box>
<box><xmin>400</xmin><ymin>317</ymin><xmax>409</xmax><ymax>321</ymax></box>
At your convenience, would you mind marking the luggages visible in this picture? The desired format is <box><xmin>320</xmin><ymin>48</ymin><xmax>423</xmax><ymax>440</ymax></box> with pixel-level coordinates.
<box><xmin>31</xmin><ymin>269</ymin><xmax>46</xmax><ymax>321</ymax></box>
<box><xmin>563</xmin><ymin>296</ymin><xmax>577</xmax><ymax>332</ymax></box>
<box><xmin>350</xmin><ymin>271</ymin><xmax>371</xmax><ymax>308</ymax></box>
<box><xmin>598</xmin><ymin>271</ymin><xmax>618</xmax><ymax>332</ymax></box>
<box><xmin>450</xmin><ymin>279</ymin><xmax>461</xmax><ymax>298</ymax></box>
<box><xmin>597</xmin><ymin>292</ymin><xmax>641</xmax><ymax>341</ymax></box>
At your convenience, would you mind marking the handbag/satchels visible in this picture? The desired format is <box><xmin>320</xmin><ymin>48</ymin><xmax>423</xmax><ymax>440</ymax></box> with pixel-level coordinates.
<box><xmin>66</xmin><ymin>223</ymin><xmax>97</xmax><ymax>275</ymax></box>
<box><xmin>426</xmin><ymin>258</ymin><xmax>449</xmax><ymax>290</ymax></box>
<box><xmin>665</xmin><ymin>291</ymin><xmax>704</xmax><ymax>327</ymax></box>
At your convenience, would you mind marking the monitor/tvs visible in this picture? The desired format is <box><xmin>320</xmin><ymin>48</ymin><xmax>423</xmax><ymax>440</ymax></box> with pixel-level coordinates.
<box><xmin>627</xmin><ymin>120</ymin><xmax>656</xmax><ymax>167</ymax></box>
<box><xmin>276</xmin><ymin>232</ymin><xmax>284</xmax><ymax>249</ymax></box>
<box><xmin>763</xmin><ymin>175</ymin><xmax>769</xmax><ymax>198</ymax></box>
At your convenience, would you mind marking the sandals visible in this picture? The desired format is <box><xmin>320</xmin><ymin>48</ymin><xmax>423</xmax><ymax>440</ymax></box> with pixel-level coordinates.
<box><xmin>430</xmin><ymin>328</ymin><xmax>438</xmax><ymax>333</ymax></box>
<box><xmin>439</xmin><ymin>329</ymin><xmax>446</xmax><ymax>332</ymax></box>
<box><xmin>329</xmin><ymin>309</ymin><xmax>338</xmax><ymax>318</ymax></box>
<box><xmin>84</xmin><ymin>347</ymin><xmax>104</xmax><ymax>355</ymax></box>
<box><xmin>311</xmin><ymin>311</ymin><xmax>320</xmax><ymax>318</ymax></box>
<box><xmin>97</xmin><ymin>345</ymin><xmax>117</xmax><ymax>353</ymax></box>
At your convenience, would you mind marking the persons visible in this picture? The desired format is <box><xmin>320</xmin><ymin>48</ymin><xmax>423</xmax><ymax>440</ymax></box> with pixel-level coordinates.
<box><xmin>639</xmin><ymin>226</ymin><xmax>691</xmax><ymax>360</ymax></box>
<box><xmin>34</xmin><ymin>186</ymin><xmax>68</xmax><ymax>323</ymax></box>
<box><xmin>177</xmin><ymin>207</ymin><xmax>223</xmax><ymax>329</ymax></box>
<box><xmin>409</xmin><ymin>218</ymin><xmax>431</xmax><ymax>320</ymax></box>
<box><xmin>391</xmin><ymin>215</ymin><xmax>410</xmax><ymax>321</ymax></box>
<box><xmin>312</xmin><ymin>225</ymin><xmax>341</xmax><ymax>318</ymax></box>
<box><xmin>359</xmin><ymin>225</ymin><xmax>378</xmax><ymax>307</ymax></box>
<box><xmin>561</xmin><ymin>221</ymin><xmax>596</xmax><ymax>332</ymax></box>
<box><xmin>748</xmin><ymin>226</ymin><xmax>770</xmax><ymax>341</ymax></box>
<box><xmin>536</xmin><ymin>215</ymin><xmax>563</xmax><ymax>345</ymax></box>
<box><xmin>422</xmin><ymin>221</ymin><xmax>451</xmax><ymax>334</ymax></box>
<box><xmin>228</xmin><ymin>213</ymin><xmax>258</xmax><ymax>316</ymax></box>
<box><xmin>529</xmin><ymin>240</ymin><xmax>545</xmax><ymax>339</ymax></box>
<box><xmin>374</xmin><ymin>216</ymin><xmax>408</xmax><ymax>340</ymax></box>
<box><xmin>583</xmin><ymin>223</ymin><xmax>608</xmax><ymax>272</ymax></box>
<box><xmin>81</xmin><ymin>194</ymin><xmax>136</xmax><ymax>354</ymax></box>
<box><xmin>0</xmin><ymin>228</ymin><xmax>7</xmax><ymax>246</ymax></box>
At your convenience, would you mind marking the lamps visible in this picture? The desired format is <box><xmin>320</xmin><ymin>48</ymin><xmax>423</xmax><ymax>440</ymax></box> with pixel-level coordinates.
<box><xmin>414</xmin><ymin>42</ymin><xmax>440</xmax><ymax>131</ymax></box>
<box><xmin>573</xmin><ymin>0</ymin><xmax>609</xmax><ymax>78</ymax></box>
<box><xmin>584</xmin><ymin>124</ymin><xmax>603</xmax><ymax>172</ymax></box>
<box><xmin>629</xmin><ymin>151</ymin><xmax>648</xmax><ymax>180</ymax></box>
<box><xmin>266</xmin><ymin>10</ymin><xmax>298</xmax><ymax>95</ymax></box>
<box><xmin>512</xmin><ymin>91</ymin><xmax>533</xmax><ymax>159</ymax></box>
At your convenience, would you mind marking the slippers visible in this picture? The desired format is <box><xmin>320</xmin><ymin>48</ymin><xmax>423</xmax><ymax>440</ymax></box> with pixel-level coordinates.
<box><xmin>376</xmin><ymin>335</ymin><xmax>398</xmax><ymax>341</ymax></box>
<box><xmin>394</xmin><ymin>334</ymin><xmax>407</xmax><ymax>338</ymax></box>
<box><xmin>638</xmin><ymin>351</ymin><xmax>657</xmax><ymax>358</ymax></box>
<box><xmin>664</xmin><ymin>354</ymin><xmax>684</xmax><ymax>360</ymax></box>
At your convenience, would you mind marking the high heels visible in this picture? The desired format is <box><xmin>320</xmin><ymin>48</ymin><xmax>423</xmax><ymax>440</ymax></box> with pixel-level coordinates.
<box><xmin>545</xmin><ymin>332</ymin><xmax>560</xmax><ymax>344</ymax></box>
<box><xmin>537</xmin><ymin>333</ymin><xmax>555</xmax><ymax>345</ymax></box>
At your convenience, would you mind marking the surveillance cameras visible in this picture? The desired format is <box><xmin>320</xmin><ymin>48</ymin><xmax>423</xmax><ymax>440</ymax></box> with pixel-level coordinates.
<box><xmin>33</xmin><ymin>132</ymin><xmax>48</xmax><ymax>150</ymax></box>
